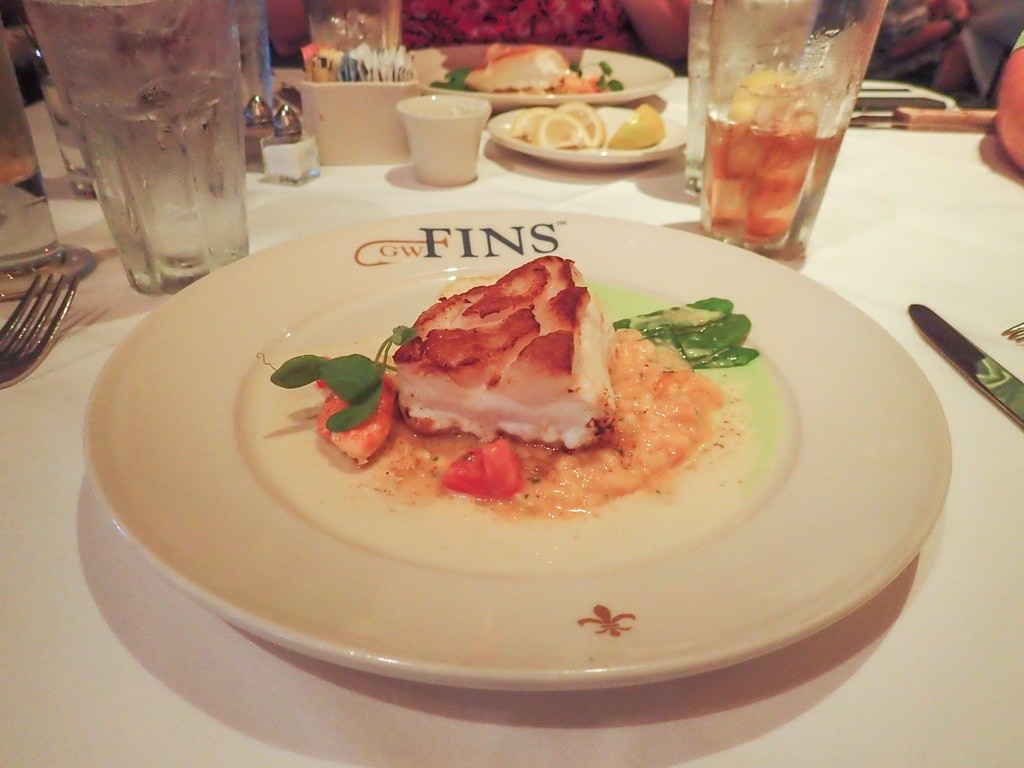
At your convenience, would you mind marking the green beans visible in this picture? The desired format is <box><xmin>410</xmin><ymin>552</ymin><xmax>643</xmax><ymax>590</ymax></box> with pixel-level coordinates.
<box><xmin>612</xmin><ymin>298</ymin><xmax>759</xmax><ymax>372</ymax></box>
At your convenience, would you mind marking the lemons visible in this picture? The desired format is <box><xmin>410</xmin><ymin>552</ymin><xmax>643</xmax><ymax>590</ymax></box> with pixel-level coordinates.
<box><xmin>721</xmin><ymin>71</ymin><xmax>792</xmax><ymax>129</ymax></box>
<box><xmin>509</xmin><ymin>101</ymin><xmax>670</xmax><ymax>151</ymax></box>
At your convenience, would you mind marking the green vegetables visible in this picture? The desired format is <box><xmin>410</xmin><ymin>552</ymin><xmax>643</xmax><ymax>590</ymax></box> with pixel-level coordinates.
<box><xmin>429</xmin><ymin>65</ymin><xmax>470</xmax><ymax>91</ymax></box>
<box><xmin>267</xmin><ymin>325</ymin><xmax>416</xmax><ymax>434</ymax></box>
<box><xmin>569</xmin><ymin>61</ymin><xmax>623</xmax><ymax>91</ymax></box>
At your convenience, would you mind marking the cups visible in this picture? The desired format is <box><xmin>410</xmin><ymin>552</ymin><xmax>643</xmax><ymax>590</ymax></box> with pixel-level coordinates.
<box><xmin>0</xmin><ymin>19</ymin><xmax>95</xmax><ymax>303</ymax></box>
<box><xmin>684</xmin><ymin>0</ymin><xmax>889</xmax><ymax>260</ymax></box>
<box><xmin>19</xmin><ymin>0</ymin><xmax>273</xmax><ymax>295</ymax></box>
<box><xmin>396</xmin><ymin>94</ymin><xmax>491</xmax><ymax>184</ymax></box>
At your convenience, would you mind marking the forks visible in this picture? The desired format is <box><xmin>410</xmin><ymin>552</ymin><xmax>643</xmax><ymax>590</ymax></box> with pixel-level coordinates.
<box><xmin>0</xmin><ymin>272</ymin><xmax>81</xmax><ymax>390</ymax></box>
<box><xmin>1003</xmin><ymin>322</ymin><xmax>1024</xmax><ymax>342</ymax></box>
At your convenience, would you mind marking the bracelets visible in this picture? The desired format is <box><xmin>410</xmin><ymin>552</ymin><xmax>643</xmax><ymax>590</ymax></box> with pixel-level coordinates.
<box><xmin>941</xmin><ymin>15</ymin><xmax>962</xmax><ymax>34</ymax></box>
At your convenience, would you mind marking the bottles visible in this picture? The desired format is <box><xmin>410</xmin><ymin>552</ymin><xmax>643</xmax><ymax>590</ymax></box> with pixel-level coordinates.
<box><xmin>242</xmin><ymin>95</ymin><xmax>277</xmax><ymax>173</ymax></box>
<box><xmin>259</xmin><ymin>105</ymin><xmax>320</xmax><ymax>186</ymax></box>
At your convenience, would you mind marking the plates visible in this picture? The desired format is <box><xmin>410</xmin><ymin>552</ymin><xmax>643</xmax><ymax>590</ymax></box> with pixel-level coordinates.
<box><xmin>407</xmin><ymin>44</ymin><xmax>675</xmax><ymax>112</ymax></box>
<box><xmin>86</xmin><ymin>209</ymin><xmax>952</xmax><ymax>692</ymax></box>
<box><xmin>486</xmin><ymin>106</ymin><xmax>687</xmax><ymax>165</ymax></box>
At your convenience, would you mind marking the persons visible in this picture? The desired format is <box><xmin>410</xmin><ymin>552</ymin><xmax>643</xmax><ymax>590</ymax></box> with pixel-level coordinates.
<box><xmin>265</xmin><ymin>1</ymin><xmax>689</xmax><ymax>60</ymax></box>
<box><xmin>864</xmin><ymin>0</ymin><xmax>1024</xmax><ymax>99</ymax></box>
<box><xmin>995</xmin><ymin>28</ymin><xmax>1024</xmax><ymax>175</ymax></box>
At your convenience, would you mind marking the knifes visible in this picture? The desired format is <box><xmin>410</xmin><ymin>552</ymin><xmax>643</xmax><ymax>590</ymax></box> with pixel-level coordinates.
<box><xmin>849</xmin><ymin>106</ymin><xmax>998</xmax><ymax>130</ymax></box>
<box><xmin>908</xmin><ymin>305</ymin><xmax>1024</xmax><ymax>424</ymax></box>
